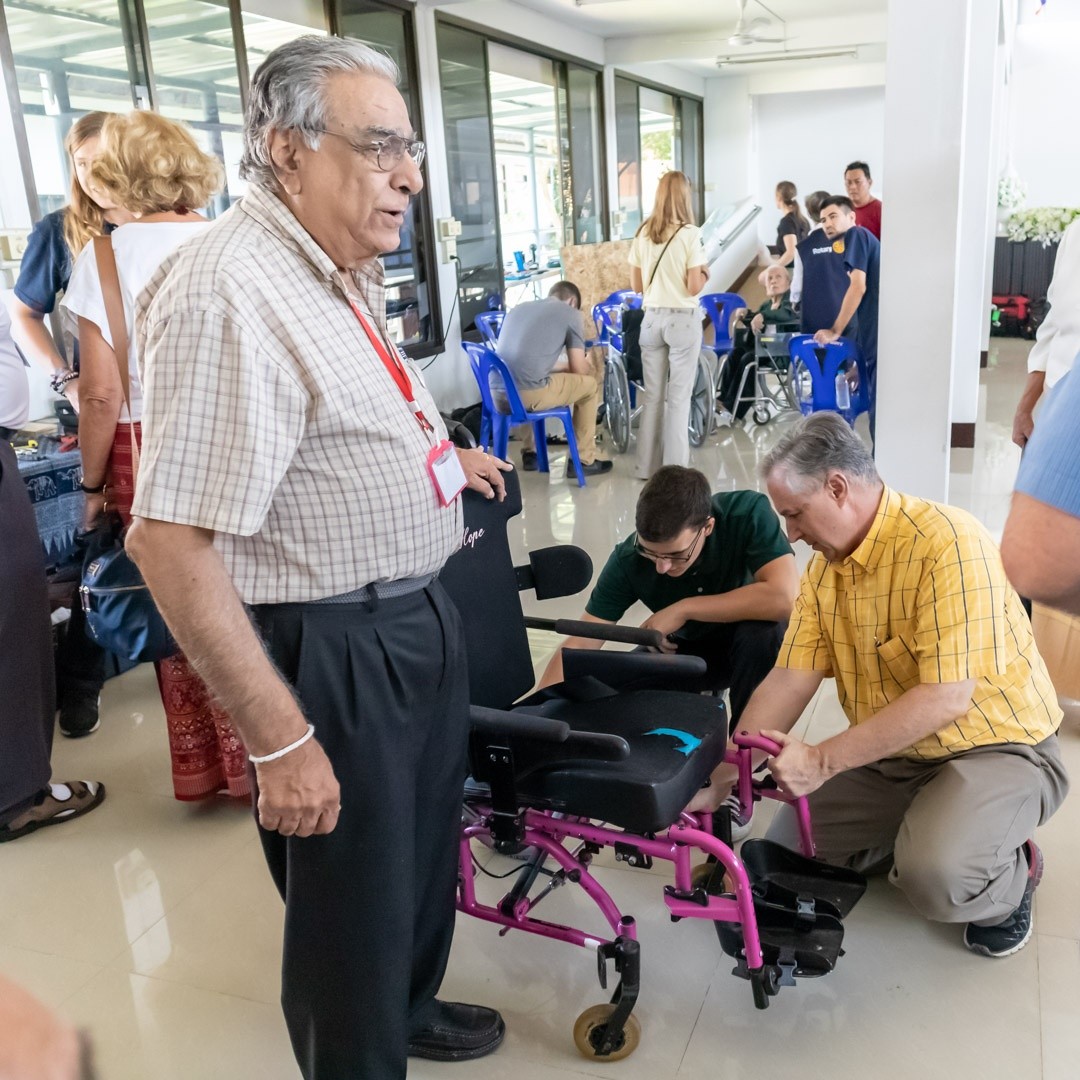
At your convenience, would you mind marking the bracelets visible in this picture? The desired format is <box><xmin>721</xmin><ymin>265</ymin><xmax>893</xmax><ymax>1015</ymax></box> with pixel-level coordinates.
<box><xmin>248</xmin><ymin>724</ymin><xmax>315</xmax><ymax>763</ymax></box>
<box><xmin>701</xmin><ymin>270</ymin><xmax>709</xmax><ymax>281</ymax></box>
<box><xmin>49</xmin><ymin>366</ymin><xmax>78</xmax><ymax>395</ymax></box>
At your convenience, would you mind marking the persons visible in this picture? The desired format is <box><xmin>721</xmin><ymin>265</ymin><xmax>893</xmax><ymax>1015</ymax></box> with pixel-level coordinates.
<box><xmin>0</xmin><ymin>36</ymin><xmax>514</xmax><ymax>1080</ymax></box>
<box><xmin>490</xmin><ymin>161</ymin><xmax>1080</xmax><ymax>957</ymax></box>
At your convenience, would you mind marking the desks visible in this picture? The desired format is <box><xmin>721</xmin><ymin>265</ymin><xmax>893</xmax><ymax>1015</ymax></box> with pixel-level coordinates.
<box><xmin>12</xmin><ymin>442</ymin><xmax>125</xmax><ymax>673</ymax></box>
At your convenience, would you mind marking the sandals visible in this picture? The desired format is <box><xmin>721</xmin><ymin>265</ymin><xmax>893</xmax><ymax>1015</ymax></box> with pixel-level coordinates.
<box><xmin>0</xmin><ymin>781</ymin><xmax>106</xmax><ymax>843</ymax></box>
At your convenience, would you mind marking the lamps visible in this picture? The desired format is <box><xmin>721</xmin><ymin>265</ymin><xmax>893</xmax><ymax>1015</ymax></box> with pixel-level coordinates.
<box><xmin>716</xmin><ymin>41</ymin><xmax>856</xmax><ymax>70</ymax></box>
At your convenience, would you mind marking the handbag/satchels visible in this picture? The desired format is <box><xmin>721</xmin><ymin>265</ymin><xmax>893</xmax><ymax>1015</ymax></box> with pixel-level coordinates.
<box><xmin>79</xmin><ymin>528</ymin><xmax>180</xmax><ymax>663</ymax></box>
<box><xmin>53</xmin><ymin>399</ymin><xmax>79</xmax><ymax>446</ymax></box>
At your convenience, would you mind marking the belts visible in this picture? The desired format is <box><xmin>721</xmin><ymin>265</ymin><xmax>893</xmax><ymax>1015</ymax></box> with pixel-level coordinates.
<box><xmin>299</xmin><ymin>569</ymin><xmax>444</xmax><ymax>605</ymax></box>
<box><xmin>0</xmin><ymin>426</ymin><xmax>18</xmax><ymax>441</ymax></box>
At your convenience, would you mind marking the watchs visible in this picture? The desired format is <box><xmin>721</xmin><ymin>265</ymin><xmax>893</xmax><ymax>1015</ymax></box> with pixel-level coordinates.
<box><xmin>80</xmin><ymin>477</ymin><xmax>105</xmax><ymax>492</ymax></box>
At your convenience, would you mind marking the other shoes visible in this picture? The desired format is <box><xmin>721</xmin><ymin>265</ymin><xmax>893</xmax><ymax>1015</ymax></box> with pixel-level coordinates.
<box><xmin>714</xmin><ymin>397</ymin><xmax>731</xmax><ymax>417</ymax></box>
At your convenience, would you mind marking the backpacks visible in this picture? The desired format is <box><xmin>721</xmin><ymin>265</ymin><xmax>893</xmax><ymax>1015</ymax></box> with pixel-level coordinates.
<box><xmin>1024</xmin><ymin>297</ymin><xmax>1051</xmax><ymax>340</ymax></box>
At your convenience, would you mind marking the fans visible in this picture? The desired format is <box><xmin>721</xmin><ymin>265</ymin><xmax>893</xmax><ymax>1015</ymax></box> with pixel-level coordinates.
<box><xmin>682</xmin><ymin>0</ymin><xmax>789</xmax><ymax>47</ymax></box>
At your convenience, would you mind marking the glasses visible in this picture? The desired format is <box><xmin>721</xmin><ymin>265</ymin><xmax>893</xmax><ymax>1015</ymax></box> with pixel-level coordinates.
<box><xmin>290</xmin><ymin>123</ymin><xmax>426</xmax><ymax>172</ymax></box>
<box><xmin>633</xmin><ymin>518</ymin><xmax>712</xmax><ymax>564</ymax></box>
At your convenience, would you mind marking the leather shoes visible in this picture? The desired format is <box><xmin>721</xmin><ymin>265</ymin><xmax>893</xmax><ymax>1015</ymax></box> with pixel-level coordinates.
<box><xmin>407</xmin><ymin>1001</ymin><xmax>505</xmax><ymax>1061</ymax></box>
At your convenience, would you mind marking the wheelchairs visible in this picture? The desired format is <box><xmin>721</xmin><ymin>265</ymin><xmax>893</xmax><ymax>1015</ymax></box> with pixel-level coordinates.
<box><xmin>600</xmin><ymin>308</ymin><xmax>717</xmax><ymax>455</ymax></box>
<box><xmin>712</xmin><ymin>314</ymin><xmax>803</xmax><ymax>427</ymax></box>
<box><xmin>436</xmin><ymin>460</ymin><xmax>868</xmax><ymax>1063</ymax></box>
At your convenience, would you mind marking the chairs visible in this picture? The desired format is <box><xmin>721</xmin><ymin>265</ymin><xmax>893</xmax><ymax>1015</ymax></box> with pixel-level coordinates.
<box><xmin>460</xmin><ymin>290</ymin><xmax>874</xmax><ymax>489</ymax></box>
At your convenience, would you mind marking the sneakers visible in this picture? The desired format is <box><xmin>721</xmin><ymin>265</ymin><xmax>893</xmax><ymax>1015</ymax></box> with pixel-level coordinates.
<box><xmin>720</xmin><ymin>791</ymin><xmax>758</xmax><ymax>842</ymax></box>
<box><xmin>521</xmin><ymin>448</ymin><xmax>538</xmax><ymax>471</ymax></box>
<box><xmin>59</xmin><ymin>683</ymin><xmax>101</xmax><ymax>738</ymax></box>
<box><xmin>567</xmin><ymin>454</ymin><xmax>613</xmax><ymax>478</ymax></box>
<box><xmin>964</xmin><ymin>838</ymin><xmax>1045</xmax><ymax>957</ymax></box>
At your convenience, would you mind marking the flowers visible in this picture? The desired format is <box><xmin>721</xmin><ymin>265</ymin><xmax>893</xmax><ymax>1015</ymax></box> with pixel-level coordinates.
<box><xmin>1005</xmin><ymin>208</ymin><xmax>1079</xmax><ymax>247</ymax></box>
